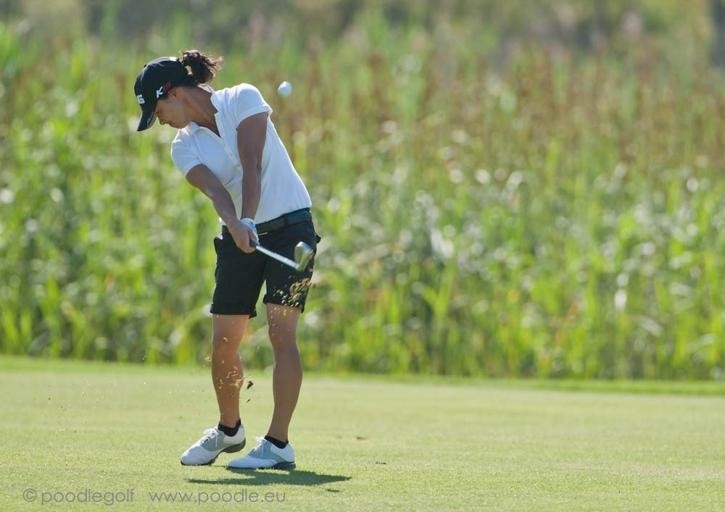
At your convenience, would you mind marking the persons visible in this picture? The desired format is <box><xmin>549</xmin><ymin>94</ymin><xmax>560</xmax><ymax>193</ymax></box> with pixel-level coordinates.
<box><xmin>133</xmin><ymin>49</ymin><xmax>321</xmax><ymax>471</ymax></box>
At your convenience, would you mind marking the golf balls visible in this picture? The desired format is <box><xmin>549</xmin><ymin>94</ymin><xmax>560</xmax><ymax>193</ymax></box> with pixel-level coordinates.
<box><xmin>276</xmin><ymin>80</ymin><xmax>294</xmax><ymax>98</ymax></box>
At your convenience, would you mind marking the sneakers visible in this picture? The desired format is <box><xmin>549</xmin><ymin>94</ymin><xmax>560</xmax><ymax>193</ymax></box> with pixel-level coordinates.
<box><xmin>226</xmin><ymin>438</ymin><xmax>297</xmax><ymax>470</ymax></box>
<box><xmin>179</xmin><ymin>423</ymin><xmax>246</xmax><ymax>466</ymax></box>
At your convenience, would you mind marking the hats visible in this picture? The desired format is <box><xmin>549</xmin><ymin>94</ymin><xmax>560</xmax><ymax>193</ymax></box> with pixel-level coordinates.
<box><xmin>134</xmin><ymin>57</ymin><xmax>188</xmax><ymax>132</ymax></box>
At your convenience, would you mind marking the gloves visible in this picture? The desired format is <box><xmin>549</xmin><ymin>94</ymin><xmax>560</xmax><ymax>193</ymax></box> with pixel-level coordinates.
<box><xmin>239</xmin><ymin>217</ymin><xmax>260</xmax><ymax>246</ymax></box>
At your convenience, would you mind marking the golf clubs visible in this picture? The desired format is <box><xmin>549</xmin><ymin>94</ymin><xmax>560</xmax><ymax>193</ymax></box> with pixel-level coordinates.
<box><xmin>247</xmin><ymin>239</ymin><xmax>314</xmax><ymax>273</ymax></box>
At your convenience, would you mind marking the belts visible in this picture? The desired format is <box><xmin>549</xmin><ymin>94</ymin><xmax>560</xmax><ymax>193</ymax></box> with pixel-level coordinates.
<box><xmin>221</xmin><ymin>210</ymin><xmax>311</xmax><ymax>234</ymax></box>
